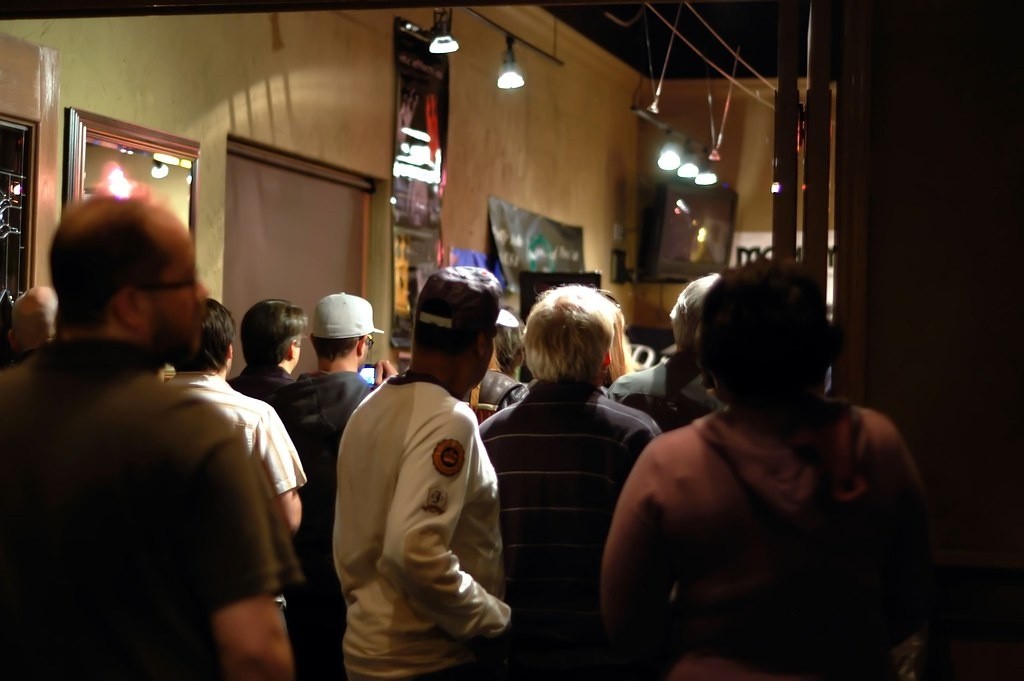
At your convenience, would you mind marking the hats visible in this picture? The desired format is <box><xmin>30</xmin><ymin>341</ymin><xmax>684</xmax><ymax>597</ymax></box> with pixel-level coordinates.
<box><xmin>312</xmin><ymin>292</ymin><xmax>385</xmax><ymax>338</ymax></box>
<box><xmin>415</xmin><ymin>266</ymin><xmax>519</xmax><ymax>328</ymax></box>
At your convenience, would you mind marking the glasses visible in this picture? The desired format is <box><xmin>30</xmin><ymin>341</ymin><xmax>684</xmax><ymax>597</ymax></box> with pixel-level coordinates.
<box><xmin>134</xmin><ymin>270</ymin><xmax>198</xmax><ymax>292</ymax></box>
<box><xmin>359</xmin><ymin>336</ymin><xmax>374</xmax><ymax>349</ymax></box>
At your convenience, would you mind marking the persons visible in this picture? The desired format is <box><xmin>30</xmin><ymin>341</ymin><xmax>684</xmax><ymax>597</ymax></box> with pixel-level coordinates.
<box><xmin>332</xmin><ymin>266</ymin><xmax>518</xmax><ymax>680</ymax></box>
<box><xmin>263</xmin><ymin>290</ymin><xmax>399</xmax><ymax>681</ymax></box>
<box><xmin>479</xmin><ymin>283</ymin><xmax>664</xmax><ymax>680</ymax></box>
<box><xmin>225</xmin><ymin>298</ymin><xmax>307</xmax><ymax>405</ymax></box>
<box><xmin>0</xmin><ymin>288</ymin><xmax>58</xmax><ymax>367</ymax></box>
<box><xmin>462</xmin><ymin>305</ymin><xmax>529</xmax><ymax>425</ymax></box>
<box><xmin>583</xmin><ymin>287</ymin><xmax>633</xmax><ymax>389</ymax></box>
<box><xmin>601</xmin><ymin>260</ymin><xmax>937</xmax><ymax>681</ymax></box>
<box><xmin>162</xmin><ymin>296</ymin><xmax>308</xmax><ymax>535</ymax></box>
<box><xmin>607</xmin><ymin>271</ymin><xmax>730</xmax><ymax>434</ymax></box>
<box><xmin>0</xmin><ymin>196</ymin><xmax>303</xmax><ymax>681</ymax></box>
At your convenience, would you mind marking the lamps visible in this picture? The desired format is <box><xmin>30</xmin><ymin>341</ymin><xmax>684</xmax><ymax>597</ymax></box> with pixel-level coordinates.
<box><xmin>428</xmin><ymin>8</ymin><xmax>459</xmax><ymax>54</ymax></box>
<box><xmin>656</xmin><ymin>134</ymin><xmax>718</xmax><ymax>185</ymax></box>
<box><xmin>497</xmin><ymin>35</ymin><xmax>525</xmax><ymax>90</ymax></box>
